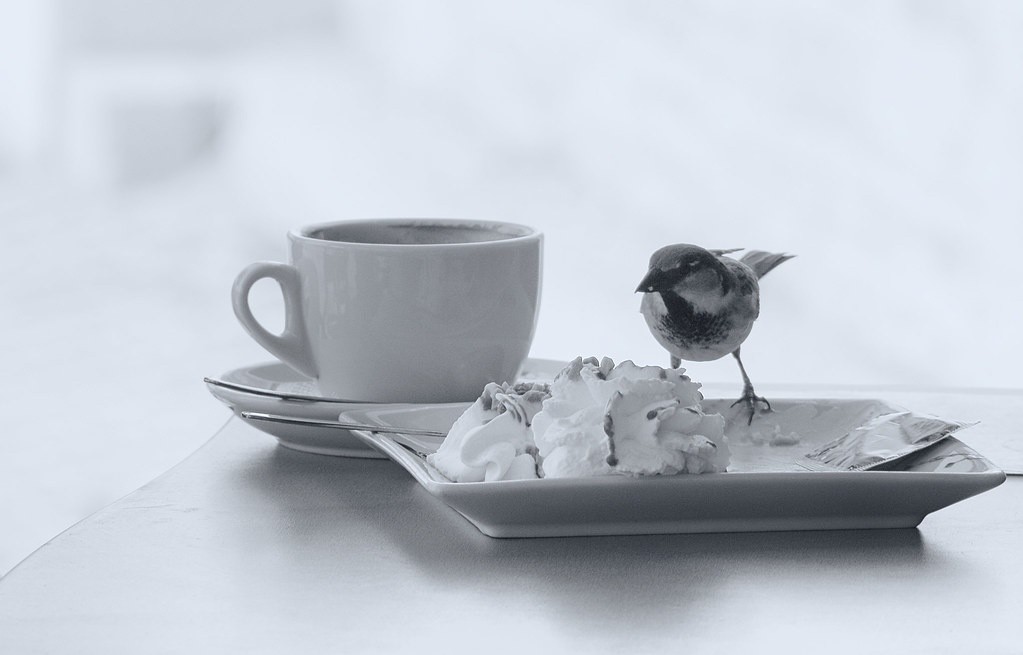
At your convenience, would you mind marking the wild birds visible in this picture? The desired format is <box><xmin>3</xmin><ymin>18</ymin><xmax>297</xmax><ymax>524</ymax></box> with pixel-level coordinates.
<box><xmin>634</xmin><ymin>243</ymin><xmax>798</xmax><ymax>426</ymax></box>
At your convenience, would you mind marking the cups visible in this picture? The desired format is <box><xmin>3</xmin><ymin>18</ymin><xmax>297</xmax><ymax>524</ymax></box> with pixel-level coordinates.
<box><xmin>230</xmin><ymin>217</ymin><xmax>543</xmax><ymax>403</ymax></box>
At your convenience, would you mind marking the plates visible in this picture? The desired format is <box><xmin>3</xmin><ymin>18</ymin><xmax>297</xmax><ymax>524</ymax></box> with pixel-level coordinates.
<box><xmin>206</xmin><ymin>356</ymin><xmax>572</xmax><ymax>459</ymax></box>
<box><xmin>340</xmin><ymin>397</ymin><xmax>1006</xmax><ymax>541</ymax></box>
